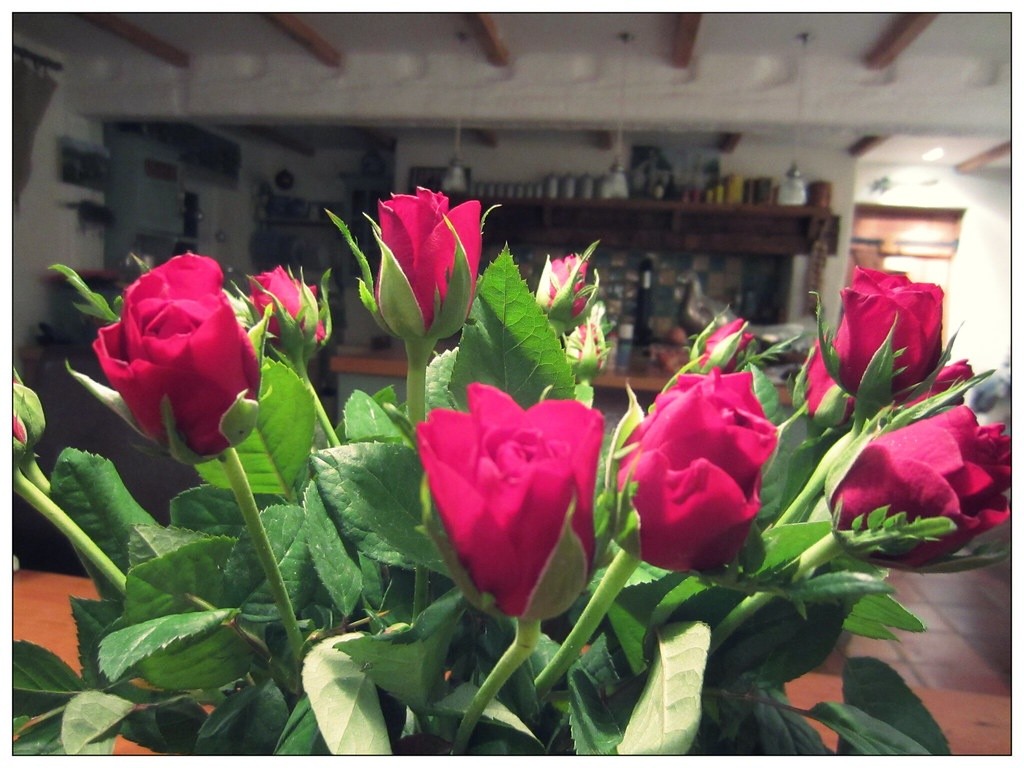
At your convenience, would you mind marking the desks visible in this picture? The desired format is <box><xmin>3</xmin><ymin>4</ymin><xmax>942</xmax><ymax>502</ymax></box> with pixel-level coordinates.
<box><xmin>11</xmin><ymin>565</ymin><xmax>1011</xmax><ymax>756</ymax></box>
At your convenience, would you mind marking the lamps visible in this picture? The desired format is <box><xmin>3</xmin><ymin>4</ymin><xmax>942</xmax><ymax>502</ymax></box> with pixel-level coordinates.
<box><xmin>442</xmin><ymin>27</ymin><xmax>471</xmax><ymax>193</ymax></box>
<box><xmin>600</xmin><ymin>31</ymin><xmax>636</xmax><ymax>199</ymax></box>
<box><xmin>775</xmin><ymin>31</ymin><xmax>814</xmax><ymax>205</ymax></box>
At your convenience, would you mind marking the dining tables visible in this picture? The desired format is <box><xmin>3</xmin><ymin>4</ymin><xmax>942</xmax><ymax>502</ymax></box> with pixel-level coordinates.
<box><xmin>12</xmin><ymin>184</ymin><xmax>1011</xmax><ymax>757</ymax></box>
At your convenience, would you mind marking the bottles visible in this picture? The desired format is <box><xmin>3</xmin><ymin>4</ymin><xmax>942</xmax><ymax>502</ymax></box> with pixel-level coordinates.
<box><xmin>580</xmin><ymin>172</ymin><xmax>598</xmax><ymax>200</ymax></box>
<box><xmin>564</xmin><ymin>169</ymin><xmax>578</xmax><ymax>200</ymax></box>
<box><xmin>630</xmin><ymin>152</ymin><xmax>773</xmax><ymax>205</ymax></box>
<box><xmin>601</xmin><ymin>156</ymin><xmax>629</xmax><ymax>200</ymax></box>
<box><xmin>468</xmin><ymin>182</ymin><xmax>545</xmax><ymax>200</ymax></box>
<box><xmin>546</xmin><ymin>172</ymin><xmax>559</xmax><ymax>200</ymax></box>
<box><xmin>776</xmin><ymin>160</ymin><xmax>834</xmax><ymax>207</ymax></box>
